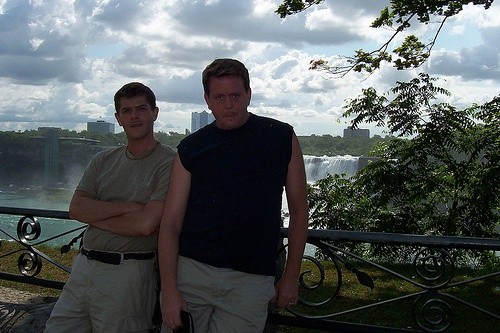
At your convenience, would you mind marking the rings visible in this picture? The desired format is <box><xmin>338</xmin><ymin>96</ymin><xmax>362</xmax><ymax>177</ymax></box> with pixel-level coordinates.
<box><xmin>288</xmin><ymin>302</ymin><xmax>293</xmax><ymax>305</ymax></box>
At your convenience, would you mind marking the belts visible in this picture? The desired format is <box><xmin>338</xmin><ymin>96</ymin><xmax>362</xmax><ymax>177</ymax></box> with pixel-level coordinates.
<box><xmin>80</xmin><ymin>247</ymin><xmax>156</xmax><ymax>265</ymax></box>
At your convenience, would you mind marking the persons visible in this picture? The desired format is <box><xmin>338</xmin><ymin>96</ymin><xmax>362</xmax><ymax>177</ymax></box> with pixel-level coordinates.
<box><xmin>157</xmin><ymin>57</ymin><xmax>309</xmax><ymax>332</ymax></box>
<box><xmin>43</xmin><ymin>82</ymin><xmax>178</xmax><ymax>333</ymax></box>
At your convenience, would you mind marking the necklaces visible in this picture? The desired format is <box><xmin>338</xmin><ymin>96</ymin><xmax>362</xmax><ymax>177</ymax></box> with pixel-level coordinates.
<box><xmin>125</xmin><ymin>142</ymin><xmax>160</xmax><ymax>162</ymax></box>
